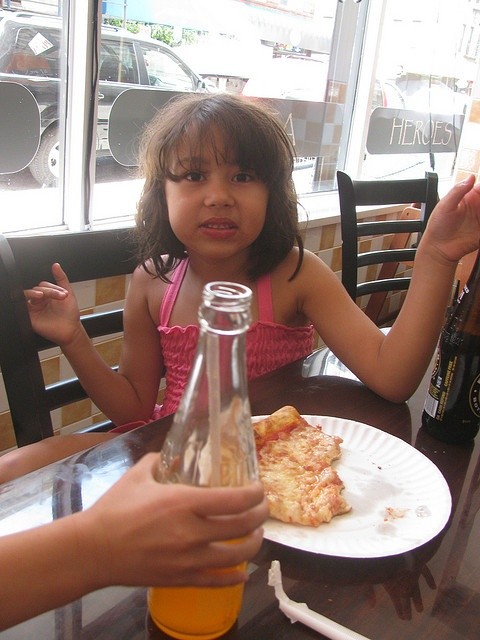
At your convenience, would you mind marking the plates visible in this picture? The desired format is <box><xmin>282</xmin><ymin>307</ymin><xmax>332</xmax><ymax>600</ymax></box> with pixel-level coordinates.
<box><xmin>198</xmin><ymin>413</ymin><xmax>454</xmax><ymax>559</ymax></box>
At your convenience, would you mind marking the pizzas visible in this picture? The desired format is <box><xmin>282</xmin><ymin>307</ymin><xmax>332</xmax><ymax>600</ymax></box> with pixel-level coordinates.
<box><xmin>211</xmin><ymin>405</ymin><xmax>354</xmax><ymax>528</ymax></box>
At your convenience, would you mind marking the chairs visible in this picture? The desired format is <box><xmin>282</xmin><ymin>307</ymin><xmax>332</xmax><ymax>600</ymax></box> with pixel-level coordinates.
<box><xmin>1</xmin><ymin>228</ymin><xmax>186</xmax><ymax>448</ymax></box>
<box><xmin>338</xmin><ymin>170</ymin><xmax>440</xmax><ymax>331</ymax></box>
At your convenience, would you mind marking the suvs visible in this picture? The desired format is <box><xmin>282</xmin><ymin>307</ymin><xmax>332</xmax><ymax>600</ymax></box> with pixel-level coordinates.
<box><xmin>1</xmin><ymin>11</ymin><xmax>225</xmax><ymax>189</ymax></box>
<box><xmin>241</xmin><ymin>55</ymin><xmax>409</xmax><ymax>163</ymax></box>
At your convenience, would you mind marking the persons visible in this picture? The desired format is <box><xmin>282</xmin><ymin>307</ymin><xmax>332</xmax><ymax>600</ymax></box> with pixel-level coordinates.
<box><xmin>25</xmin><ymin>88</ymin><xmax>480</xmax><ymax>482</ymax></box>
<box><xmin>2</xmin><ymin>452</ymin><xmax>271</xmax><ymax>630</ymax></box>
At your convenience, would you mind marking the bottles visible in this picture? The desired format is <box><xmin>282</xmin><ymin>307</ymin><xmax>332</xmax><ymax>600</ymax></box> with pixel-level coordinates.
<box><xmin>146</xmin><ymin>281</ymin><xmax>260</xmax><ymax>640</ymax></box>
<box><xmin>419</xmin><ymin>239</ymin><xmax>480</xmax><ymax>446</ymax></box>
